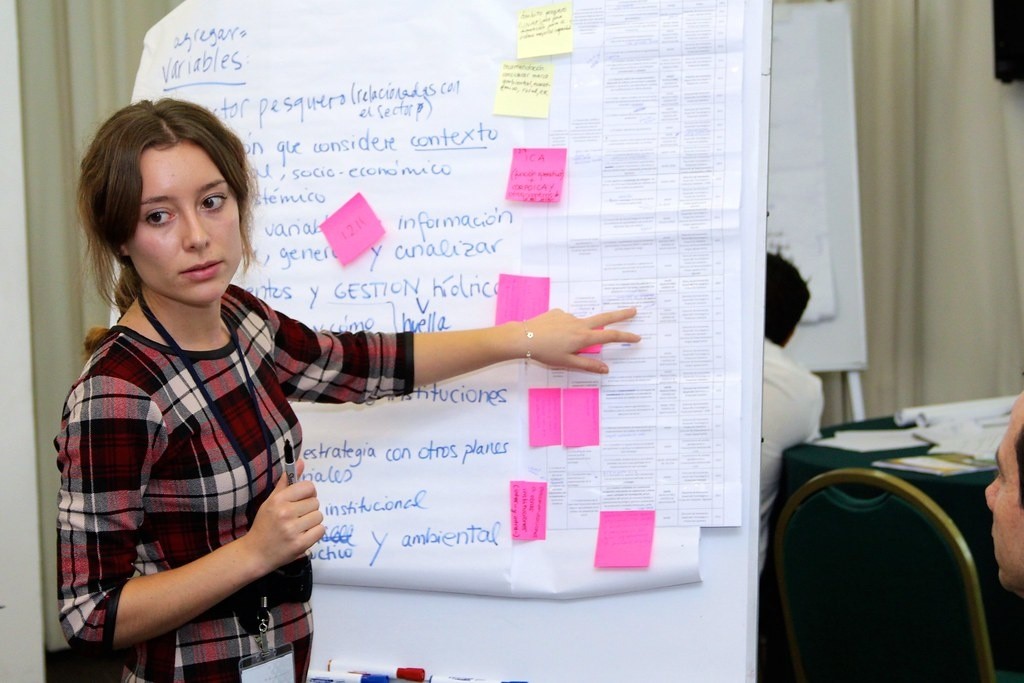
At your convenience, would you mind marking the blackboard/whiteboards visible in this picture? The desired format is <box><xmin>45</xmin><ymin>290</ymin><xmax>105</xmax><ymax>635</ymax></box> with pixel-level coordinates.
<box><xmin>764</xmin><ymin>1</ymin><xmax>871</xmax><ymax>373</ymax></box>
<box><xmin>128</xmin><ymin>0</ymin><xmax>777</xmax><ymax>683</ymax></box>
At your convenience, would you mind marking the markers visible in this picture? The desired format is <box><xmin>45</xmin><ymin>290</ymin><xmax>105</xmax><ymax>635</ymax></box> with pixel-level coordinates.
<box><xmin>305</xmin><ymin>657</ymin><xmax>427</xmax><ymax>683</ymax></box>
<box><xmin>282</xmin><ymin>438</ymin><xmax>298</xmax><ymax>486</ymax></box>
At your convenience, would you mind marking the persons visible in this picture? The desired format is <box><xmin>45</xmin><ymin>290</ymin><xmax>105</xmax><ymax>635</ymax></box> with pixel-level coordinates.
<box><xmin>54</xmin><ymin>99</ymin><xmax>640</xmax><ymax>683</ymax></box>
<box><xmin>984</xmin><ymin>371</ymin><xmax>1024</xmax><ymax>598</ymax></box>
<box><xmin>759</xmin><ymin>252</ymin><xmax>825</xmax><ymax>683</ymax></box>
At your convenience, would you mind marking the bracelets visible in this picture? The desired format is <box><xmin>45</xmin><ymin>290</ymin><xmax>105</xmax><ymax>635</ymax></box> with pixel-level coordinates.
<box><xmin>523</xmin><ymin>319</ymin><xmax>534</xmax><ymax>364</ymax></box>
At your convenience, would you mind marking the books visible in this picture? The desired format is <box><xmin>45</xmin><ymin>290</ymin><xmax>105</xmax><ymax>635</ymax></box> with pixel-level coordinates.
<box><xmin>874</xmin><ymin>453</ymin><xmax>997</xmax><ymax>476</ymax></box>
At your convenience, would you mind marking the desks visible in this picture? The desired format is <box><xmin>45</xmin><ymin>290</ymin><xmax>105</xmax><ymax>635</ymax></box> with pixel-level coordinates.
<box><xmin>758</xmin><ymin>395</ymin><xmax>1024</xmax><ymax>683</ymax></box>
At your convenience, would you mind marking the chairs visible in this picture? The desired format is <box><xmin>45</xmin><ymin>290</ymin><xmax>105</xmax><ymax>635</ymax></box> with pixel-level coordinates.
<box><xmin>759</xmin><ymin>466</ymin><xmax>998</xmax><ymax>683</ymax></box>
